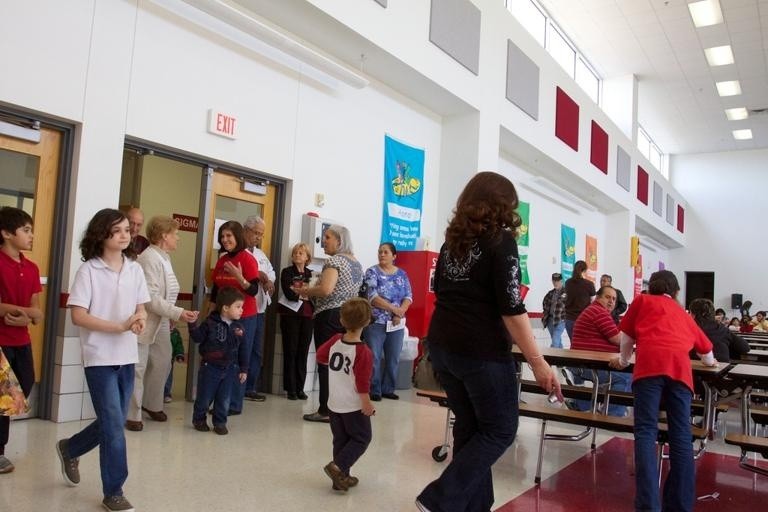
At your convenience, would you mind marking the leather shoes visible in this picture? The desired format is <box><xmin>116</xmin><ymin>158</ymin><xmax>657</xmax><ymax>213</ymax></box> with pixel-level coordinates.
<box><xmin>287</xmin><ymin>389</ymin><xmax>308</xmax><ymax>401</ymax></box>
<box><xmin>303</xmin><ymin>412</ymin><xmax>331</xmax><ymax>423</ymax></box>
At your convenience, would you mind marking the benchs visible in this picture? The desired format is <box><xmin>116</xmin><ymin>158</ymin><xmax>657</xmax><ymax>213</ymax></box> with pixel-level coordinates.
<box><xmin>411</xmin><ymin>320</ymin><xmax>767</xmax><ymax>503</ymax></box>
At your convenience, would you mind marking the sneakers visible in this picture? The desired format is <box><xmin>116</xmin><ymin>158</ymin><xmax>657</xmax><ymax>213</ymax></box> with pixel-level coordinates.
<box><xmin>141</xmin><ymin>406</ymin><xmax>168</xmax><ymax>422</ymax></box>
<box><xmin>125</xmin><ymin>419</ymin><xmax>143</xmax><ymax>431</ymax></box>
<box><xmin>193</xmin><ymin>390</ymin><xmax>265</xmax><ymax>435</ymax></box>
<box><xmin>325</xmin><ymin>461</ymin><xmax>358</xmax><ymax>492</ymax></box>
<box><xmin>57</xmin><ymin>439</ymin><xmax>80</xmax><ymax>486</ymax></box>
<box><xmin>382</xmin><ymin>390</ymin><xmax>399</xmax><ymax>400</ymax></box>
<box><xmin>0</xmin><ymin>454</ymin><xmax>15</xmax><ymax>472</ymax></box>
<box><xmin>371</xmin><ymin>394</ymin><xmax>381</xmax><ymax>401</ymax></box>
<box><xmin>102</xmin><ymin>496</ymin><xmax>135</xmax><ymax>512</ymax></box>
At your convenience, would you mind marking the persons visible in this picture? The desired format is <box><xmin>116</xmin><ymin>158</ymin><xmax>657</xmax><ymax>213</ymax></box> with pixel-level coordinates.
<box><xmin>1</xmin><ymin>207</ymin><xmax>45</xmax><ymax>474</ymax></box>
<box><xmin>688</xmin><ymin>300</ymin><xmax>750</xmax><ymax>435</ymax></box>
<box><xmin>609</xmin><ymin>269</ymin><xmax>720</xmax><ymax>511</ymax></box>
<box><xmin>126</xmin><ymin>208</ymin><xmax>363</xmax><ymax>433</ymax></box>
<box><xmin>55</xmin><ymin>208</ymin><xmax>153</xmax><ymax>510</ymax></box>
<box><xmin>316</xmin><ymin>298</ymin><xmax>376</xmax><ymax>491</ymax></box>
<box><xmin>361</xmin><ymin>242</ymin><xmax>412</xmax><ymax>401</ymax></box>
<box><xmin>409</xmin><ymin>170</ymin><xmax>565</xmax><ymax>512</ymax></box>
<box><xmin>715</xmin><ymin>300</ymin><xmax>768</xmax><ymax>334</ymax></box>
<box><xmin>541</xmin><ymin>259</ymin><xmax>632</xmax><ymax>417</ymax></box>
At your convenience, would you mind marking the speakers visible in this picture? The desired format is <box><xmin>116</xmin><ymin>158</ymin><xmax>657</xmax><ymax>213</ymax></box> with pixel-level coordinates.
<box><xmin>731</xmin><ymin>294</ymin><xmax>742</xmax><ymax>309</ymax></box>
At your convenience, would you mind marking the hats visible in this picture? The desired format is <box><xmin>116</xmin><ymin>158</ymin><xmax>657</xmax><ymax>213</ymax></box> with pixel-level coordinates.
<box><xmin>552</xmin><ymin>273</ymin><xmax>562</xmax><ymax>281</ymax></box>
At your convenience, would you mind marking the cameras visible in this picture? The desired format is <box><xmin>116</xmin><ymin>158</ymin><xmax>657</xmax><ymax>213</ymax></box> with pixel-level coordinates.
<box><xmin>548</xmin><ymin>386</ymin><xmax>564</xmax><ymax>403</ymax></box>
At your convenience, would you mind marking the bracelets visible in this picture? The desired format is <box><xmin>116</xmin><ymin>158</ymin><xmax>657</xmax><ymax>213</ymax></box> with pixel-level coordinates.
<box><xmin>523</xmin><ymin>352</ymin><xmax>545</xmax><ymax>370</ymax></box>
